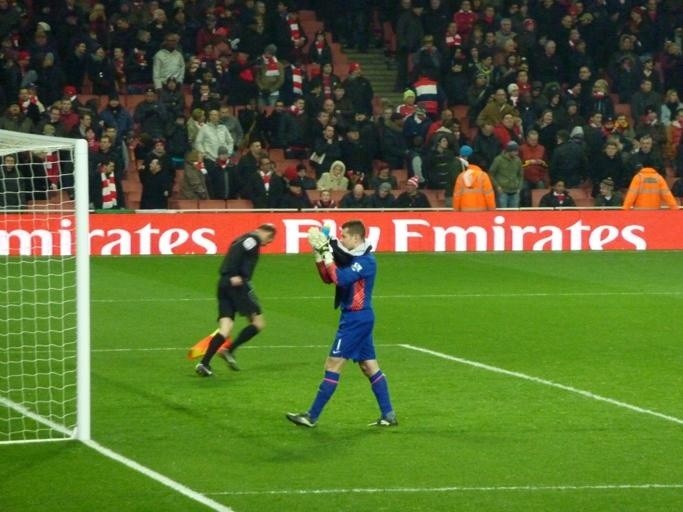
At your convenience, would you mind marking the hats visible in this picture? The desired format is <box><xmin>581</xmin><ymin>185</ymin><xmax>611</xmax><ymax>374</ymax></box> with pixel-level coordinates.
<box><xmin>218</xmin><ymin>146</ymin><xmax>227</xmax><ymax>155</ymax></box>
<box><xmin>644</xmin><ymin>104</ymin><xmax>658</xmax><ymax>115</ymax></box>
<box><xmin>38</xmin><ymin>21</ymin><xmax>51</xmax><ymax>31</ymax></box>
<box><xmin>403</xmin><ymin>87</ymin><xmax>415</xmax><ymax>101</ymax></box>
<box><xmin>409</xmin><ymin>175</ymin><xmax>420</xmax><ymax>189</ymax></box>
<box><xmin>460</xmin><ymin>145</ymin><xmax>473</xmax><ymax>156</ymax></box>
<box><xmin>64</xmin><ymin>86</ymin><xmax>76</xmax><ymax>95</ymax></box>
<box><xmin>109</xmin><ymin>93</ymin><xmax>118</xmax><ymax>101</ymax></box>
<box><xmin>348</xmin><ymin>62</ymin><xmax>360</xmax><ymax>75</ymax></box>
<box><xmin>265</xmin><ymin>44</ymin><xmax>277</xmax><ymax>54</ymax></box>
<box><xmin>639</xmin><ymin>52</ymin><xmax>651</xmax><ymax>64</ymax></box>
<box><xmin>523</xmin><ymin>19</ymin><xmax>536</xmax><ymax>29</ymax></box>
<box><xmin>592</xmin><ymin>79</ymin><xmax>608</xmax><ymax>96</ymax></box>
<box><xmin>193</xmin><ymin>108</ymin><xmax>205</xmax><ymax>121</ymax></box>
<box><xmin>633</xmin><ymin>7</ymin><xmax>642</xmax><ymax>15</ymax></box>
<box><xmin>19</xmin><ymin>51</ymin><xmax>30</xmax><ymax>60</ymax></box>
<box><xmin>415</xmin><ymin>103</ymin><xmax>425</xmax><ymax>116</ymax></box>
<box><xmin>508</xmin><ymin>83</ymin><xmax>519</xmax><ymax>94</ymax></box>
<box><xmin>146</xmin><ymin>76</ymin><xmax>176</xmax><ymax>92</ymax></box>
<box><xmin>507</xmin><ymin>140</ymin><xmax>518</xmax><ymax>151</ymax></box>
<box><xmin>580</xmin><ymin>13</ymin><xmax>593</xmax><ymax>21</ymax></box>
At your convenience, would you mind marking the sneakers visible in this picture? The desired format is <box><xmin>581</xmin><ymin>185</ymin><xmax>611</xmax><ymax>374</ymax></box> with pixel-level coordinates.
<box><xmin>368</xmin><ymin>418</ymin><xmax>399</xmax><ymax>427</ymax></box>
<box><xmin>220</xmin><ymin>350</ymin><xmax>240</xmax><ymax>370</ymax></box>
<box><xmin>195</xmin><ymin>362</ymin><xmax>213</xmax><ymax>377</ymax></box>
<box><xmin>286</xmin><ymin>412</ymin><xmax>318</xmax><ymax>427</ymax></box>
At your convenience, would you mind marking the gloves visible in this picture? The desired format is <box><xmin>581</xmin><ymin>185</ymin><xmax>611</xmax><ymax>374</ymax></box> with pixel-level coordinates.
<box><xmin>308</xmin><ymin>223</ymin><xmax>330</xmax><ymax>258</ymax></box>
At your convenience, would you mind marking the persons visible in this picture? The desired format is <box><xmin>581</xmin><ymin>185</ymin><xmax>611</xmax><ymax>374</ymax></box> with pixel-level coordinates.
<box><xmin>193</xmin><ymin>223</ymin><xmax>279</xmax><ymax>376</ymax></box>
<box><xmin>282</xmin><ymin>218</ymin><xmax>401</xmax><ymax>429</ymax></box>
<box><xmin>0</xmin><ymin>0</ymin><xmax>683</xmax><ymax>213</ymax></box>
